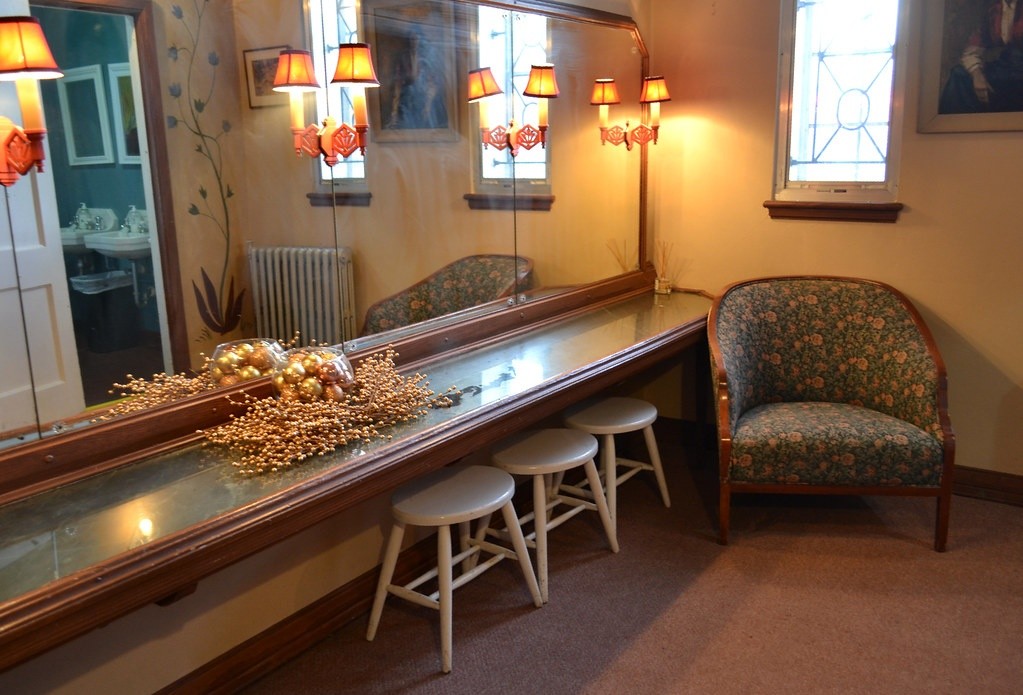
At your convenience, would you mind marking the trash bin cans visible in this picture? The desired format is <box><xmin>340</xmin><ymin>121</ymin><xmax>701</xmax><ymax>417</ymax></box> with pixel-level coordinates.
<box><xmin>70</xmin><ymin>270</ymin><xmax>137</xmax><ymax>353</ymax></box>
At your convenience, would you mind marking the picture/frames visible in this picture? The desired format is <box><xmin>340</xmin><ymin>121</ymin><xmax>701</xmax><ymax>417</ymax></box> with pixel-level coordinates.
<box><xmin>107</xmin><ymin>62</ymin><xmax>141</xmax><ymax>164</ymax></box>
<box><xmin>356</xmin><ymin>0</ymin><xmax>460</xmax><ymax>144</ymax></box>
<box><xmin>55</xmin><ymin>65</ymin><xmax>115</xmax><ymax>165</ymax></box>
<box><xmin>242</xmin><ymin>45</ymin><xmax>293</xmax><ymax>109</ymax></box>
<box><xmin>916</xmin><ymin>0</ymin><xmax>1023</xmax><ymax>133</ymax></box>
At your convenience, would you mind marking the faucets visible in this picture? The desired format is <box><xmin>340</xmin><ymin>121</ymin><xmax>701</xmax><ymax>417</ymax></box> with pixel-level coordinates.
<box><xmin>84</xmin><ymin>220</ymin><xmax>92</xmax><ymax>230</ymax></box>
<box><xmin>69</xmin><ymin>215</ymin><xmax>80</xmax><ymax>229</ymax></box>
<box><xmin>138</xmin><ymin>221</ymin><xmax>145</xmax><ymax>233</ymax></box>
<box><xmin>120</xmin><ymin>217</ymin><xmax>131</xmax><ymax>233</ymax></box>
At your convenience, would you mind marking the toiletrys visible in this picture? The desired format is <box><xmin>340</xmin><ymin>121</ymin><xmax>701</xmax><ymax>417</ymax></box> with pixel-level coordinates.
<box><xmin>125</xmin><ymin>205</ymin><xmax>144</xmax><ymax>233</ymax></box>
<box><xmin>77</xmin><ymin>202</ymin><xmax>93</xmax><ymax>230</ymax></box>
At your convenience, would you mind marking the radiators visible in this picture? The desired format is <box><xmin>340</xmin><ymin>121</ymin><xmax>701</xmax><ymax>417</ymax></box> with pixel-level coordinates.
<box><xmin>247</xmin><ymin>244</ymin><xmax>358</xmax><ymax>351</ymax></box>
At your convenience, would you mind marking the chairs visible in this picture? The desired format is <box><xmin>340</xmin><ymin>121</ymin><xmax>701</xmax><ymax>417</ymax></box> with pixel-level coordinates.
<box><xmin>707</xmin><ymin>275</ymin><xmax>956</xmax><ymax>552</ymax></box>
<box><xmin>363</xmin><ymin>253</ymin><xmax>536</xmax><ymax>337</ymax></box>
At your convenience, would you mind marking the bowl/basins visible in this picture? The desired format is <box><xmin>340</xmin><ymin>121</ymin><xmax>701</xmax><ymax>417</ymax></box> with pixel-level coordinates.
<box><xmin>208</xmin><ymin>337</ymin><xmax>282</xmax><ymax>388</ymax></box>
<box><xmin>271</xmin><ymin>347</ymin><xmax>354</xmax><ymax>406</ymax></box>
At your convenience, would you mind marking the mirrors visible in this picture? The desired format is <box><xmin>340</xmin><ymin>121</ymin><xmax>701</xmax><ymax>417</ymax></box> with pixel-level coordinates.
<box><xmin>0</xmin><ymin>0</ymin><xmax>649</xmax><ymax>485</ymax></box>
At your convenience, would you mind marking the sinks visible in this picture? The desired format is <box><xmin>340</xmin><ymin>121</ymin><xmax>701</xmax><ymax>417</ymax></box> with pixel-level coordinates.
<box><xmin>83</xmin><ymin>210</ymin><xmax>152</xmax><ymax>260</ymax></box>
<box><xmin>60</xmin><ymin>208</ymin><xmax>119</xmax><ymax>254</ymax></box>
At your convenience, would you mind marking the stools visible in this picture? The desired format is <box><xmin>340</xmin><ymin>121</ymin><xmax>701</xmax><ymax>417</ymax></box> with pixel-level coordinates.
<box><xmin>552</xmin><ymin>397</ymin><xmax>671</xmax><ymax>532</ymax></box>
<box><xmin>364</xmin><ymin>464</ymin><xmax>543</xmax><ymax>674</ymax></box>
<box><xmin>468</xmin><ymin>428</ymin><xmax>619</xmax><ymax>604</ymax></box>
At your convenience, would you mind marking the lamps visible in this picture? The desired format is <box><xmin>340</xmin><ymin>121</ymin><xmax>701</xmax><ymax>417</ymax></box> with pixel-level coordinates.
<box><xmin>319</xmin><ymin>43</ymin><xmax>381</xmax><ymax>168</ymax></box>
<box><xmin>0</xmin><ymin>16</ymin><xmax>65</xmax><ymax>191</ymax></box>
<box><xmin>624</xmin><ymin>75</ymin><xmax>672</xmax><ymax>151</ymax></box>
<box><xmin>508</xmin><ymin>65</ymin><xmax>560</xmax><ymax>157</ymax></box>
<box><xmin>590</xmin><ymin>78</ymin><xmax>624</xmax><ymax>147</ymax></box>
<box><xmin>272</xmin><ymin>49</ymin><xmax>325</xmax><ymax>159</ymax></box>
<box><xmin>467</xmin><ymin>67</ymin><xmax>511</xmax><ymax>150</ymax></box>
